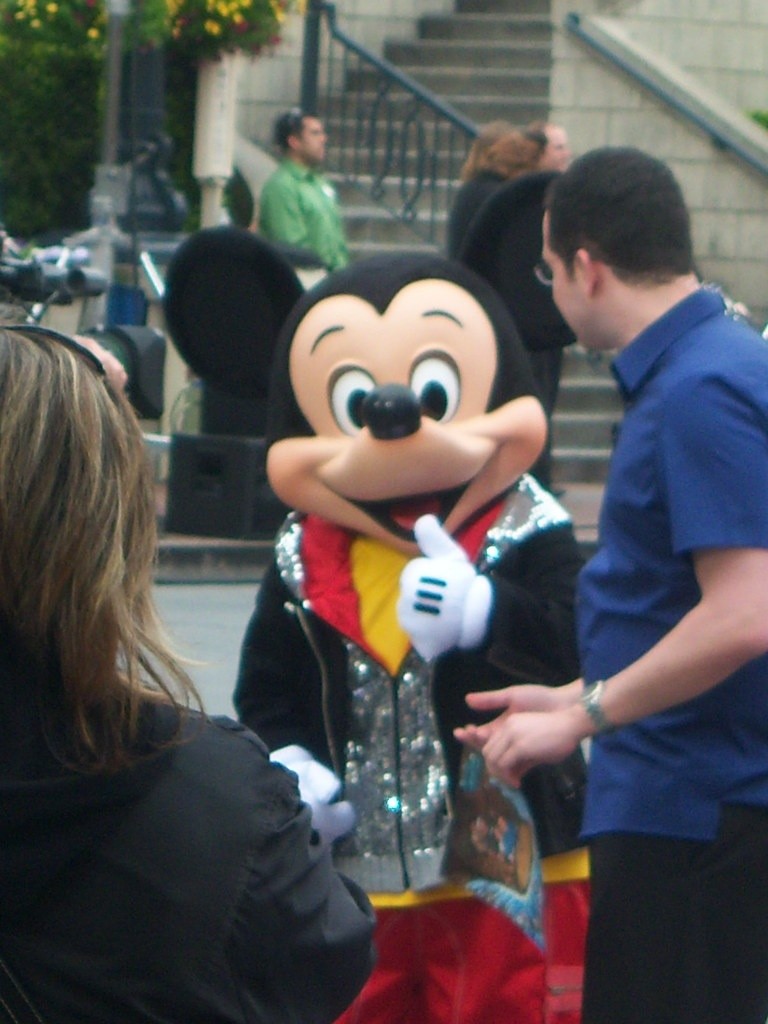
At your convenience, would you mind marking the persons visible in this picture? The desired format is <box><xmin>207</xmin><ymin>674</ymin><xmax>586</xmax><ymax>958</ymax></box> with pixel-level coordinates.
<box><xmin>0</xmin><ymin>313</ymin><xmax>378</xmax><ymax>1024</ymax></box>
<box><xmin>452</xmin><ymin>142</ymin><xmax>768</xmax><ymax>1024</ymax></box>
<box><xmin>448</xmin><ymin>120</ymin><xmax>570</xmax><ymax>502</ymax></box>
<box><xmin>256</xmin><ymin>106</ymin><xmax>348</xmax><ymax>291</ymax></box>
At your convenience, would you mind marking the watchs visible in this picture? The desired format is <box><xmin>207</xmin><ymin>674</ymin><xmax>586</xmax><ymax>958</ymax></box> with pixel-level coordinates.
<box><xmin>581</xmin><ymin>680</ymin><xmax>613</xmax><ymax>734</ymax></box>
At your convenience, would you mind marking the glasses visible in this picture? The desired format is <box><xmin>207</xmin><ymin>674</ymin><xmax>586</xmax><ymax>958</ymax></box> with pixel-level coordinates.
<box><xmin>534</xmin><ymin>248</ymin><xmax>584</xmax><ymax>287</ymax></box>
<box><xmin>0</xmin><ymin>322</ymin><xmax>117</xmax><ymax>405</ymax></box>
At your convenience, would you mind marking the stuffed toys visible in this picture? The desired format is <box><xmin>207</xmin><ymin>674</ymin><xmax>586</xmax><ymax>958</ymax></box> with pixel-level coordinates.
<box><xmin>164</xmin><ymin>169</ymin><xmax>589</xmax><ymax>1024</ymax></box>
